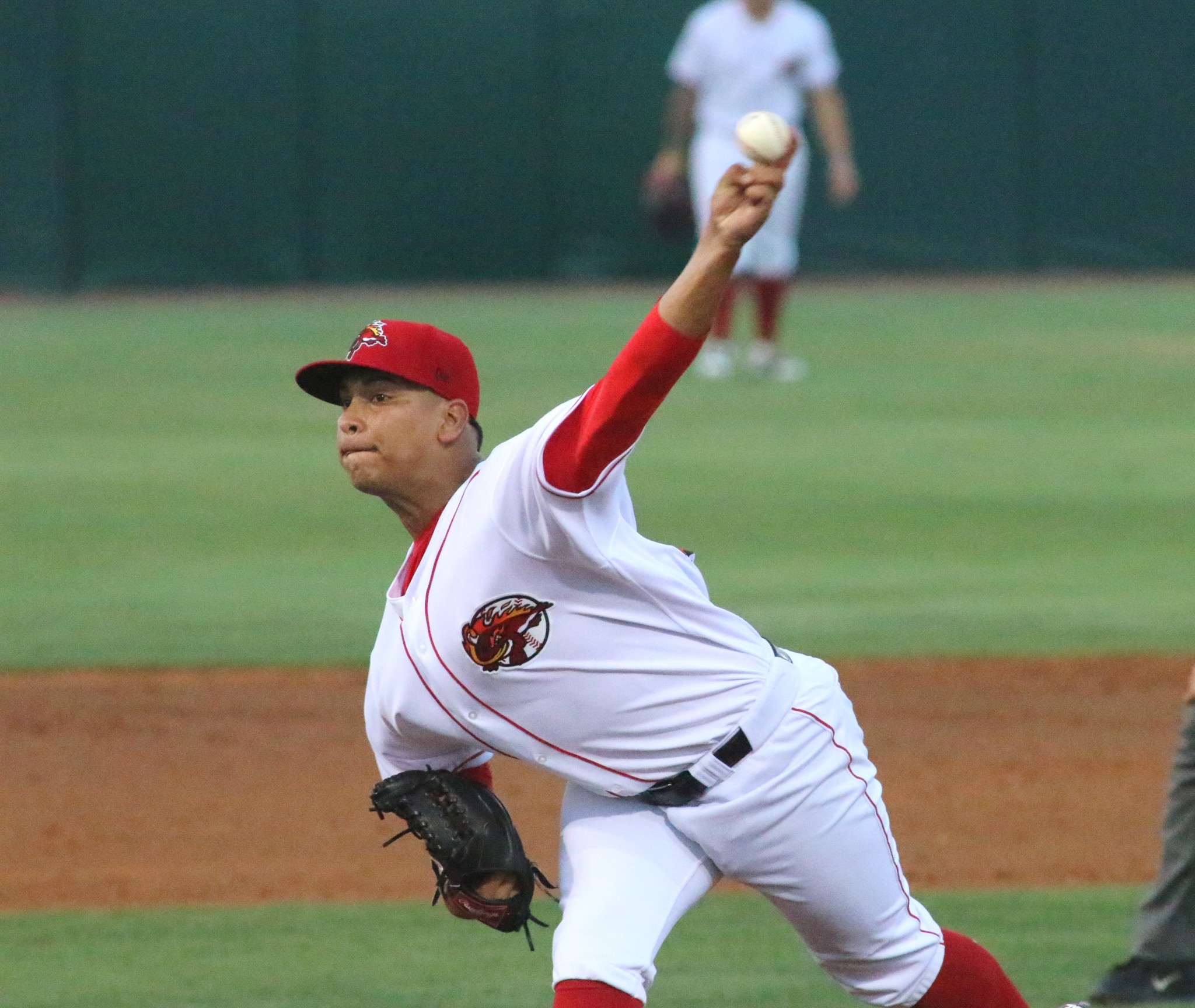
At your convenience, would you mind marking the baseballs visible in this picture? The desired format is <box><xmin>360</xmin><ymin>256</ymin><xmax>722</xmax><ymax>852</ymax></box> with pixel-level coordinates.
<box><xmin>735</xmin><ymin>114</ymin><xmax>796</xmax><ymax>159</ymax></box>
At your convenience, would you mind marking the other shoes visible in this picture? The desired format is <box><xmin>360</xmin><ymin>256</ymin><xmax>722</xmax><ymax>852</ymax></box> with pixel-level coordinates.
<box><xmin>702</xmin><ymin>348</ymin><xmax>729</xmax><ymax>374</ymax></box>
<box><xmin>751</xmin><ymin>340</ymin><xmax>779</xmax><ymax>375</ymax></box>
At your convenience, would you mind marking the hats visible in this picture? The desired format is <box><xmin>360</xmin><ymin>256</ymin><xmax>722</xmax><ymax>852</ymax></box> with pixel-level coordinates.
<box><xmin>294</xmin><ymin>318</ymin><xmax>481</xmax><ymax>422</ymax></box>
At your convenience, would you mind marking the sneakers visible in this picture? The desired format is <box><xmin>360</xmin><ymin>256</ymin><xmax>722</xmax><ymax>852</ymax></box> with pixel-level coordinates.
<box><xmin>1063</xmin><ymin>956</ymin><xmax>1195</xmax><ymax>1008</ymax></box>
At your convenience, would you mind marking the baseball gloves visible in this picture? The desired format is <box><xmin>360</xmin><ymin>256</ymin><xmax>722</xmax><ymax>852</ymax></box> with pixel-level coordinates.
<box><xmin>368</xmin><ymin>771</ymin><xmax>533</xmax><ymax>933</ymax></box>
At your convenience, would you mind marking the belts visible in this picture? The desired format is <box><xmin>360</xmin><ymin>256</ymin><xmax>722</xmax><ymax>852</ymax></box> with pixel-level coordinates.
<box><xmin>639</xmin><ymin>636</ymin><xmax>793</xmax><ymax>807</ymax></box>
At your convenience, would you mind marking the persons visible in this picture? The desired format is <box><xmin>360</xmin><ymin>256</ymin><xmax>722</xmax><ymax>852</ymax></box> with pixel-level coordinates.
<box><xmin>639</xmin><ymin>0</ymin><xmax>860</xmax><ymax>384</ymax></box>
<box><xmin>292</xmin><ymin>130</ymin><xmax>1030</xmax><ymax>1008</ymax></box>
<box><xmin>1063</xmin><ymin>666</ymin><xmax>1195</xmax><ymax>1008</ymax></box>
